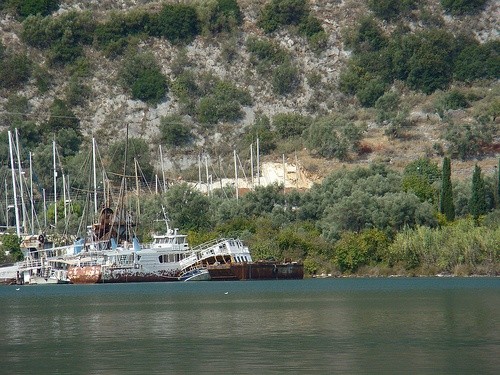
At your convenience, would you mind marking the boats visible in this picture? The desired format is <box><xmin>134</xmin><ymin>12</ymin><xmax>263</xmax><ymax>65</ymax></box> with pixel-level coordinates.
<box><xmin>14</xmin><ymin>227</ymin><xmax>304</xmax><ymax>283</ymax></box>
